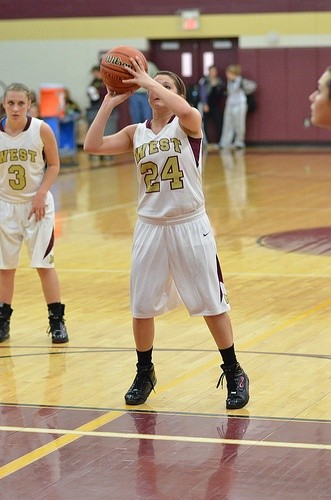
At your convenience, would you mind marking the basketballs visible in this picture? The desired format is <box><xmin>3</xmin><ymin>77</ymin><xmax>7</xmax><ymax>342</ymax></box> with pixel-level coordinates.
<box><xmin>100</xmin><ymin>45</ymin><xmax>148</xmax><ymax>93</ymax></box>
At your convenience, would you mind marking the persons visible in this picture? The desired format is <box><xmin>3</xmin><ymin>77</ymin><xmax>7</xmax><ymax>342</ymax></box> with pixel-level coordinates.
<box><xmin>84</xmin><ymin>56</ymin><xmax>249</xmax><ymax>410</ymax></box>
<box><xmin>86</xmin><ymin>65</ymin><xmax>258</xmax><ymax>168</ymax></box>
<box><xmin>0</xmin><ymin>83</ymin><xmax>69</xmax><ymax>343</ymax></box>
<box><xmin>308</xmin><ymin>67</ymin><xmax>331</xmax><ymax>129</ymax></box>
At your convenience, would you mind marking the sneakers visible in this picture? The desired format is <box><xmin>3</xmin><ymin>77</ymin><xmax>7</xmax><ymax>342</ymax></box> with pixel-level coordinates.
<box><xmin>124</xmin><ymin>362</ymin><xmax>157</xmax><ymax>406</ymax></box>
<box><xmin>0</xmin><ymin>303</ymin><xmax>13</xmax><ymax>342</ymax></box>
<box><xmin>47</xmin><ymin>302</ymin><xmax>69</xmax><ymax>343</ymax></box>
<box><xmin>216</xmin><ymin>362</ymin><xmax>249</xmax><ymax>409</ymax></box>
